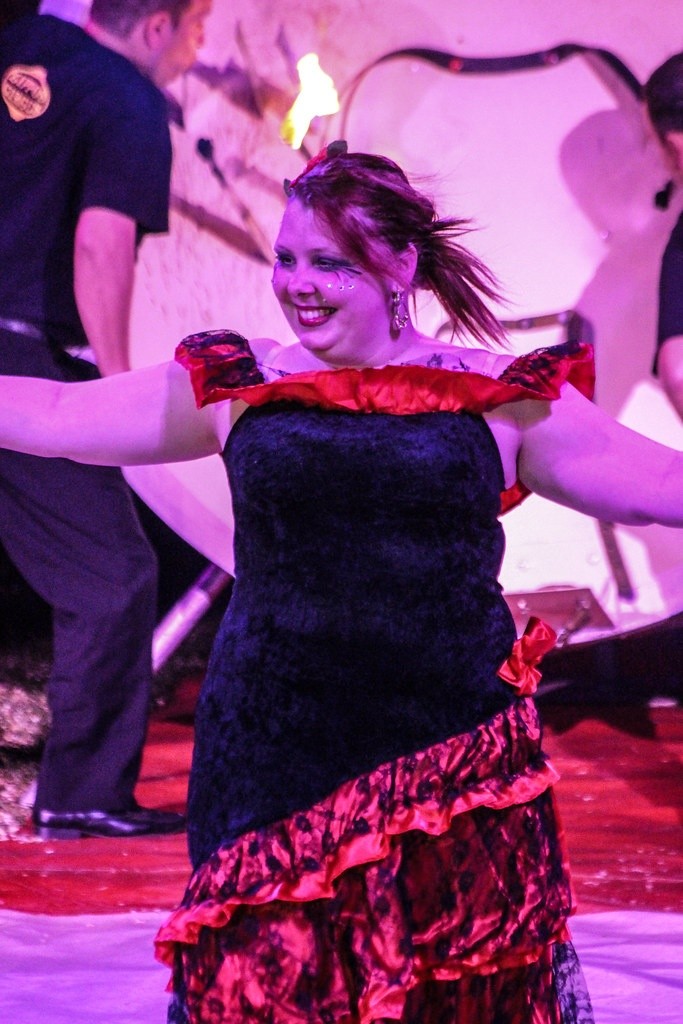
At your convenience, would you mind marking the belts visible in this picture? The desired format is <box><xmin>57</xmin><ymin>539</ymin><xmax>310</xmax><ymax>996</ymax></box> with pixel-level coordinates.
<box><xmin>0</xmin><ymin>317</ymin><xmax>43</xmax><ymax>342</ymax></box>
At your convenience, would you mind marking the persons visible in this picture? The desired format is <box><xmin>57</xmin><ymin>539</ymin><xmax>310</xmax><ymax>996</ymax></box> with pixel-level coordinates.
<box><xmin>0</xmin><ymin>0</ymin><xmax>213</xmax><ymax>838</ymax></box>
<box><xmin>648</xmin><ymin>51</ymin><xmax>683</xmax><ymax>427</ymax></box>
<box><xmin>0</xmin><ymin>138</ymin><xmax>683</xmax><ymax>1024</ymax></box>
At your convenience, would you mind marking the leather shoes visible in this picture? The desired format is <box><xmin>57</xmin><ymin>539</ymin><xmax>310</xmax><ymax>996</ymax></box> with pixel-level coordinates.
<box><xmin>33</xmin><ymin>805</ymin><xmax>184</xmax><ymax>840</ymax></box>
